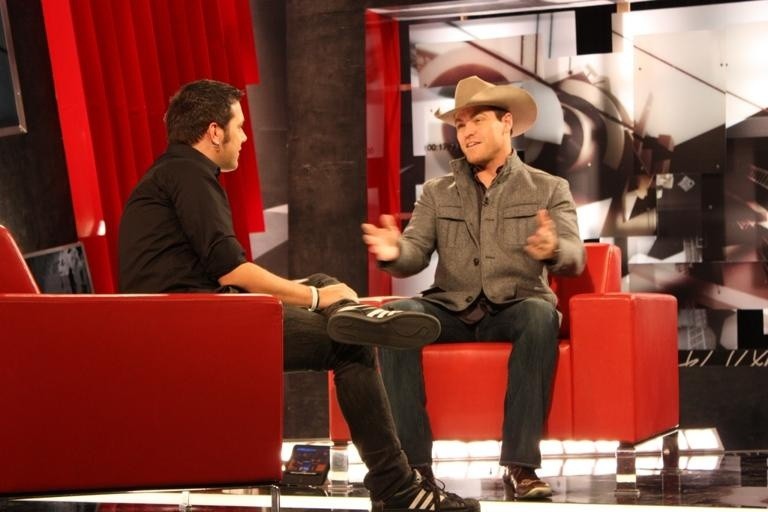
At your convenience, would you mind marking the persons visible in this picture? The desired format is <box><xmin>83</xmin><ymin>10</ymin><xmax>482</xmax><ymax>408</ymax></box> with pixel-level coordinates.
<box><xmin>118</xmin><ymin>79</ymin><xmax>481</xmax><ymax>512</ymax></box>
<box><xmin>361</xmin><ymin>74</ymin><xmax>585</xmax><ymax>501</ymax></box>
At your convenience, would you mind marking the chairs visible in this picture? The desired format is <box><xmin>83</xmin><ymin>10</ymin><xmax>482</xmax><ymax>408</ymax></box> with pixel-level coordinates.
<box><xmin>330</xmin><ymin>242</ymin><xmax>678</xmax><ymax>481</ymax></box>
<box><xmin>1</xmin><ymin>226</ymin><xmax>284</xmax><ymax>512</ymax></box>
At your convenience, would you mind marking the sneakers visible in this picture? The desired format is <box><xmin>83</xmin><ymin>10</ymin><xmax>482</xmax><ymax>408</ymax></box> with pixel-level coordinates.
<box><xmin>372</xmin><ymin>469</ymin><xmax>481</xmax><ymax>511</ymax></box>
<box><xmin>327</xmin><ymin>304</ymin><xmax>441</xmax><ymax>351</ymax></box>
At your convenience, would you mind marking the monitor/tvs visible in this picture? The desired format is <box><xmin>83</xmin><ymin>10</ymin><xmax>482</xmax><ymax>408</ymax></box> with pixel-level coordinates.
<box><xmin>398</xmin><ymin>0</ymin><xmax>768</xmax><ymax>366</ymax></box>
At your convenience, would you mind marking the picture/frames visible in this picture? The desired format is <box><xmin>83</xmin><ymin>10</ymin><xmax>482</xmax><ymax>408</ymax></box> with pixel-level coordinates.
<box><xmin>1</xmin><ymin>1</ymin><xmax>27</xmax><ymax>137</ymax></box>
<box><xmin>23</xmin><ymin>242</ymin><xmax>93</xmax><ymax>295</ymax></box>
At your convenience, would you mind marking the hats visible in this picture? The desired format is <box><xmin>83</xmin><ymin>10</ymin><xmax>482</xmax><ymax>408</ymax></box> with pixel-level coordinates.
<box><xmin>434</xmin><ymin>77</ymin><xmax>537</xmax><ymax>137</ymax></box>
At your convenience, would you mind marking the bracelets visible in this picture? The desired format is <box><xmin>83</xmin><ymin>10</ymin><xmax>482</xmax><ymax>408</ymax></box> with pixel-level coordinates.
<box><xmin>308</xmin><ymin>286</ymin><xmax>320</xmax><ymax>311</ymax></box>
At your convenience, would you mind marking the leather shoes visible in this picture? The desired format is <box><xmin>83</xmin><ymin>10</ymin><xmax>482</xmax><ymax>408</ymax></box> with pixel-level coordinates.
<box><xmin>503</xmin><ymin>465</ymin><xmax>552</xmax><ymax>499</ymax></box>
